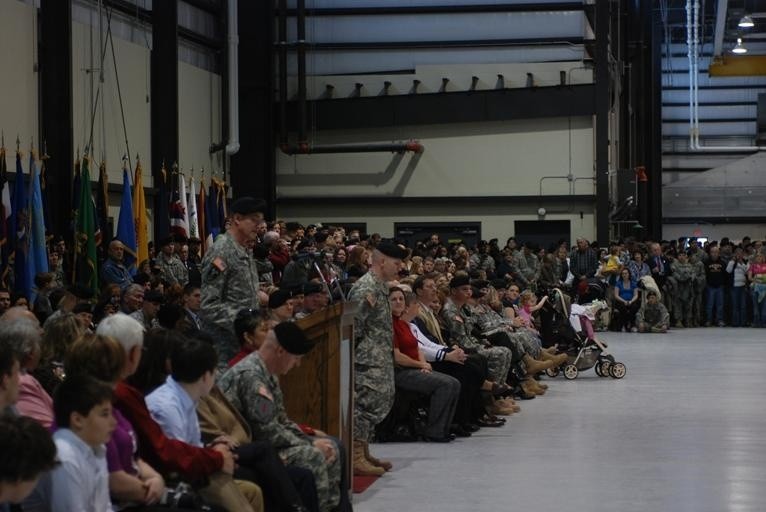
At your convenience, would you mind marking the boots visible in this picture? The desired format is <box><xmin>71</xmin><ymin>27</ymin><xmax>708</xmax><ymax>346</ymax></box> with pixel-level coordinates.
<box><xmin>524</xmin><ymin>347</ymin><xmax>567</xmax><ymax>375</ymax></box>
<box><xmin>495</xmin><ymin>378</ymin><xmax>548</xmax><ymax>415</ymax></box>
<box><xmin>352</xmin><ymin>439</ymin><xmax>392</xmax><ymax>476</ymax></box>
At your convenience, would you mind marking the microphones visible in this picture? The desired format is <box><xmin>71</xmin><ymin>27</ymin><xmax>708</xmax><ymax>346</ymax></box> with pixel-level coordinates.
<box><xmin>309</xmin><ymin>250</ymin><xmax>346</xmax><ymax>302</ymax></box>
<box><xmin>292</xmin><ymin>251</ymin><xmax>336</xmax><ymax>305</ymax></box>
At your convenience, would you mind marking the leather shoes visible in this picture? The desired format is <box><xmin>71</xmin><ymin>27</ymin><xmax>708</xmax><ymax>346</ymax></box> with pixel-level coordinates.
<box><xmin>440</xmin><ymin>413</ymin><xmax>506</xmax><ymax>442</ymax></box>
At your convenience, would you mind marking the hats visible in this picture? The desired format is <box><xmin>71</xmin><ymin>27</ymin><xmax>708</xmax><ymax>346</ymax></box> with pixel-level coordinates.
<box><xmin>449</xmin><ymin>275</ymin><xmax>507</xmax><ymax>299</ymax></box>
<box><xmin>284</xmin><ymin>277</ymin><xmax>324</xmax><ymax>297</ymax></box>
<box><xmin>231</xmin><ymin>197</ymin><xmax>268</xmax><ymax>215</ymax></box>
<box><xmin>647</xmin><ymin>291</ymin><xmax>656</xmax><ymax>299</ymax></box>
<box><xmin>143</xmin><ymin>288</ymin><xmax>166</xmax><ymax>303</ymax></box>
<box><xmin>376</xmin><ymin>237</ymin><xmax>407</xmax><ymax>259</ymax></box>
<box><xmin>269</xmin><ymin>290</ymin><xmax>293</xmax><ymax>309</ymax></box>
<box><xmin>274</xmin><ymin>321</ymin><xmax>316</xmax><ymax>356</ymax></box>
<box><xmin>71</xmin><ymin>301</ymin><xmax>92</xmax><ymax>314</ymax></box>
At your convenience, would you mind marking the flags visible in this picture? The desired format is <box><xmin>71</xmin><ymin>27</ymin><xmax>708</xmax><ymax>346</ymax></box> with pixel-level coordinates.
<box><xmin>1</xmin><ymin>131</ymin><xmax>229</xmax><ymax>308</ymax></box>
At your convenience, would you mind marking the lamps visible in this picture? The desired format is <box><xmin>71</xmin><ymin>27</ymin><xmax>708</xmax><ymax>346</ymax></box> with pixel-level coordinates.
<box><xmin>730</xmin><ymin>30</ymin><xmax>749</xmax><ymax>55</ymax></box>
<box><xmin>609</xmin><ymin>218</ymin><xmax>645</xmax><ymax>231</ymax></box>
<box><xmin>737</xmin><ymin>1</ymin><xmax>756</xmax><ymax>29</ymax></box>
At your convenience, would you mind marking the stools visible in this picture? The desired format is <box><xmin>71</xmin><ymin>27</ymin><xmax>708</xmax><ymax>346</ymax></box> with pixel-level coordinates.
<box><xmin>386</xmin><ymin>390</ymin><xmax>431</xmax><ymax>442</ymax></box>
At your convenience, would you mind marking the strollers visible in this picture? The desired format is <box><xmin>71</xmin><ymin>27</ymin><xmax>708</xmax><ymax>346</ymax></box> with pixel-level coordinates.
<box><xmin>531</xmin><ymin>280</ymin><xmax>626</xmax><ymax>380</ymax></box>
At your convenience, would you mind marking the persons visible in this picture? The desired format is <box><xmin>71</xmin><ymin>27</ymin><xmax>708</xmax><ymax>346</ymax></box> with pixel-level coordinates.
<box><xmin>1</xmin><ymin>197</ymin><xmax>765</xmax><ymax>512</ymax></box>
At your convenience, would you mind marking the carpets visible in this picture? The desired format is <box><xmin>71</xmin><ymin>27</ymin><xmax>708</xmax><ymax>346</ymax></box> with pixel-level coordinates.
<box><xmin>348</xmin><ymin>459</ymin><xmax>387</xmax><ymax>494</ymax></box>
